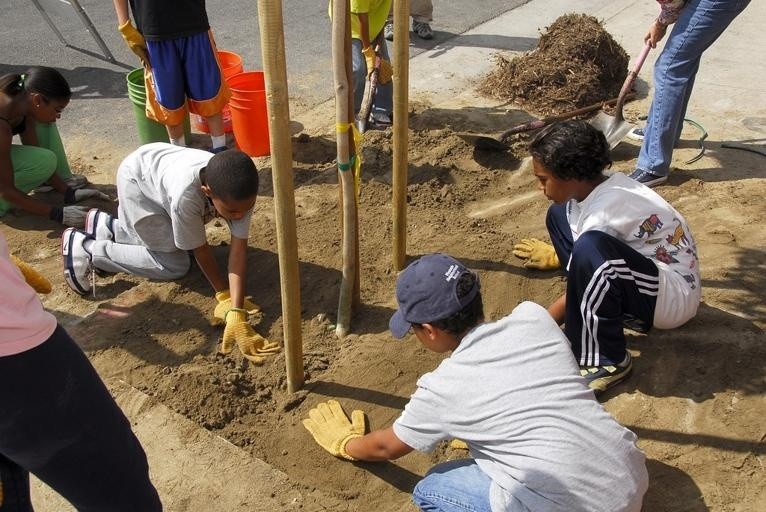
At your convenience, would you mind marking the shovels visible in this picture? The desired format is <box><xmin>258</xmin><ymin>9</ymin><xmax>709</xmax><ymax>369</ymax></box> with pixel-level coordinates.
<box><xmin>587</xmin><ymin>37</ymin><xmax>651</xmax><ymax>151</ymax></box>
<box><xmin>456</xmin><ymin>92</ymin><xmax>637</xmax><ymax>152</ymax></box>
<box><xmin>354</xmin><ymin>27</ymin><xmax>384</xmax><ymax>134</ymax></box>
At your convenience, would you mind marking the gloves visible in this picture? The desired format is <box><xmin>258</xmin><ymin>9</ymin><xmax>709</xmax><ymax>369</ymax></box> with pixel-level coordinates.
<box><xmin>360</xmin><ymin>42</ymin><xmax>393</xmax><ymax>89</ymax></box>
<box><xmin>116</xmin><ymin>13</ymin><xmax>153</xmax><ymax>71</ymax></box>
<box><xmin>206</xmin><ymin>284</ymin><xmax>257</xmax><ymax>332</ymax></box>
<box><xmin>508</xmin><ymin>236</ymin><xmax>563</xmax><ymax>275</ymax></box>
<box><xmin>68</xmin><ymin>179</ymin><xmax>108</xmax><ymax>204</ymax></box>
<box><xmin>215</xmin><ymin>304</ymin><xmax>285</xmax><ymax>369</ymax></box>
<box><xmin>298</xmin><ymin>398</ymin><xmax>373</xmax><ymax>464</ymax></box>
<box><xmin>54</xmin><ymin>207</ymin><xmax>87</xmax><ymax>225</ymax></box>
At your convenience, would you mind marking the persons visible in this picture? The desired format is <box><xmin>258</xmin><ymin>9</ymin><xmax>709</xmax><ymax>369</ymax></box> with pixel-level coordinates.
<box><xmin>510</xmin><ymin>117</ymin><xmax>701</xmax><ymax>399</ymax></box>
<box><xmin>63</xmin><ymin>142</ymin><xmax>281</xmax><ymax>364</ymax></box>
<box><xmin>628</xmin><ymin>0</ymin><xmax>754</xmax><ymax>187</ymax></box>
<box><xmin>303</xmin><ymin>253</ymin><xmax>648</xmax><ymax>512</ymax></box>
<box><xmin>114</xmin><ymin>0</ymin><xmax>228</xmax><ymax>154</ymax></box>
<box><xmin>0</xmin><ymin>65</ymin><xmax>111</xmax><ymax>230</ymax></box>
<box><xmin>384</xmin><ymin>0</ymin><xmax>436</xmax><ymax>42</ymax></box>
<box><xmin>328</xmin><ymin>0</ymin><xmax>419</xmax><ymax>131</ymax></box>
<box><xmin>0</xmin><ymin>238</ymin><xmax>163</xmax><ymax>512</ymax></box>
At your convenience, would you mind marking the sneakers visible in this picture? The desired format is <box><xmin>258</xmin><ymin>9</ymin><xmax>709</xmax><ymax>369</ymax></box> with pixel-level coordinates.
<box><xmin>368</xmin><ymin>107</ymin><xmax>395</xmax><ymax>129</ymax></box>
<box><xmin>406</xmin><ymin>16</ymin><xmax>436</xmax><ymax>42</ymax></box>
<box><xmin>80</xmin><ymin>206</ymin><xmax>118</xmax><ymax>281</ymax></box>
<box><xmin>623</xmin><ymin>163</ymin><xmax>674</xmax><ymax>193</ymax></box>
<box><xmin>382</xmin><ymin>19</ymin><xmax>396</xmax><ymax>39</ymax></box>
<box><xmin>579</xmin><ymin>349</ymin><xmax>637</xmax><ymax>400</ymax></box>
<box><xmin>56</xmin><ymin>224</ymin><xmax>95</xmax><ymax>304</ymax></box>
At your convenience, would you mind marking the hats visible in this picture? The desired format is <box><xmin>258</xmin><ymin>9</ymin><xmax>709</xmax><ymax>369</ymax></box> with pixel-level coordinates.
<box><xmin>385</xmin><ymin>251</ymin><xmax>483</xmax><ymax>341</ymax></box>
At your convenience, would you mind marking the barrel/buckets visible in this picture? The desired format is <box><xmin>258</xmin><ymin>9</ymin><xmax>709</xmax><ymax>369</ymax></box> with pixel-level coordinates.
<box><xmin>126</xmin><ymin>66</ymin><xmax>192</xmax><ymax>147</ymax></box>
<box><xmin>195</xmin><ymin>49</ymin><xmax>242</xmax><ymax>134</ymax></box>
<box><xmin>224</xmin><ymin>71</ymin><xmax>270</xmax><ymax>157</ymax></box>
